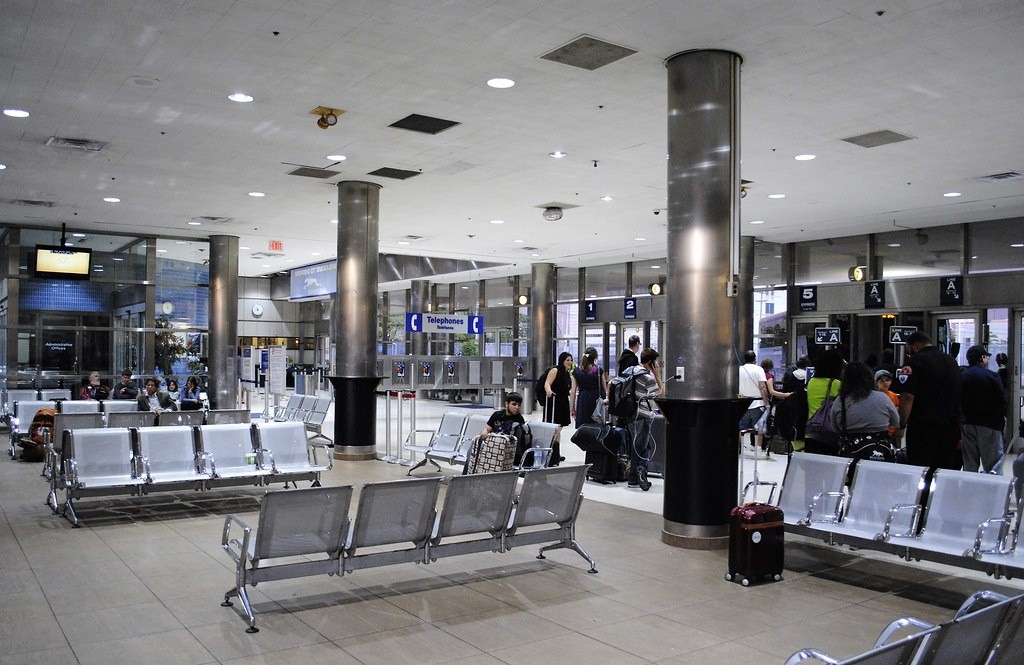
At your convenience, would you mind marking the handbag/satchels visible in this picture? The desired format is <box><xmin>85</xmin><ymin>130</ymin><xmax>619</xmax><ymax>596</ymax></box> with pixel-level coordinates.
<box><xmin>896</xmin><ymin>447</ymin><xmax>906</xmax><ymax>464</ymax></box>
<box><xmin>754</xmin><ymin>404</ymin><xmax>786</xmax><ymax>454</ymax></box>
<box><xmin>775</xmin><ymin>377</ymin><xmax>813</xmax><ymax>440</ymax></box>
<box><xmin>591</xmin><ymin>398</ymin><xmax>618</xmax><ymax>425</ymax></box>
<box><xmin>181</xmin><ymin>401</ymin><xmax>201</xmax><ymax>411</ymax></box>
<box><xmin>836</xmin><ymin>442</ymin><xmax>896</xmax><ymax>486</ymax></box>
<box><xmin>570</xmin><ymin>423</ymin><xmax>621</xmax><ymax>456</ymax></box>
<box><xmin>805</xmin><ymin>378</ymin><xmax>836</xmax><ymax>435</ymax></box>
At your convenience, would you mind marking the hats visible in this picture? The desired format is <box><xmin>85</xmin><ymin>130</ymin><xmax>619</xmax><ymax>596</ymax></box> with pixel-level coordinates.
<box><xmin>874</xmin><ymin>370</ymin><xmax>893</xmax><ymax>383</ymax></box>
<box><xmin>967</xmin><ymin>346</ymin><xmax>991</xmax><ymax>356</ymax></box>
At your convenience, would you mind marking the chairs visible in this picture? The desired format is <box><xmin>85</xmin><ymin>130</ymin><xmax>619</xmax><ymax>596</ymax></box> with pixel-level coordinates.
<box><xmin>737</xmin><ymin>453</ymin><xmax>1024</xmax><ymax>579</ymax></box>
<box><xmin>781</xmin><ymin>590</ymin><xmax>1024</xmax><ymax>665</ymax></box>
<box><xmin>3</xmin><ymin>379</ymin><xmax>599</xmax><ymax>633</ymax></box>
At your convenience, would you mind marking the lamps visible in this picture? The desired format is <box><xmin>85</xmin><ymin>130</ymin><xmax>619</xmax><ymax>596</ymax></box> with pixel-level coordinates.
<box><xmin>848</xmin><ymin>266</ymin><xmax>867</xmax><ymax>282</ymax></box>
<box><xmin>309</xmin><ymin>106</ymin><xmax>346</xmax><ymax>129</ymax></box>
<box><xmin>648</xmin><ymin>283</ymin><xmax>661</xmax><ymax>295</ymax></box>
<box><xmin>741</xmin><ymin>187</ymin><xmax>747</xmax><ymax>198</ymax></box>
<box><xmin>534</xmin><ymin>199</ymin><xmax>582</xmax><ymax>221</ymax></box>
<box><xmin>517</xmin><ymin>294</ymin><xmax>530</xmax><ymax>305</ymax></box>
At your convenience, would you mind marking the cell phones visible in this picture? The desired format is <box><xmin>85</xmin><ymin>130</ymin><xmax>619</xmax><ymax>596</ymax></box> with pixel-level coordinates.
<box><xmin>649</xmin><ymin>360</ymin><xmax>655</xmax><ymax>369</ymax></box>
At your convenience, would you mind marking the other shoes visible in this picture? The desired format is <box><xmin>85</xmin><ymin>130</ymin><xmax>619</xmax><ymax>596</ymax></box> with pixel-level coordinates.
<box><xmin>744</xmin><ymin>444</ymin><xmax>746</xmax><ymax>450</ymax></box>
<box><xmin>761</xmin><ymin>451</ymin><xmax>767</xmax><ymax>457</ymax></box>
<box><xmin>750</xmin><ymin>445</ymin><xmax>755</xmax><ymax>451</ymax></box>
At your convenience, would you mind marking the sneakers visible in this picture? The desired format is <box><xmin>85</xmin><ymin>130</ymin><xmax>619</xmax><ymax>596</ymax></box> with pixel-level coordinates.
<box><xmin>627</xmin><ymin>483</ymin><xmax>652</xmax><ymax>488</ymax></box>
<box><xmin>636</xmin><ymin>466</ymin><xmax>649</xmax><ymax>491</ymax></box>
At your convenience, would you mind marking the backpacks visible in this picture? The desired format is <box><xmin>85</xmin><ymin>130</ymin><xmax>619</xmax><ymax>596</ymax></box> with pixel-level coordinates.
<box><xmin>608</xmin><ymin>366</ymin><xmax>650</xmax><ymax>418</ymax></box>
<box><xmin>510</xmin><ymin>422</ymin><xmax>534</xmax><ymax>466</ymax></box>
<box><xmin>616</xmin><ymin>454</ymin><xmax>631</xmax><ymax>482</ymax></box>
<box><xmin>535</xmin><ymin>366</ymin><xmax>560</xmax><ymax>406</ymax></box>
<box><xmin>89</xmin><ymin>384</ymin><xmax>109</xmax><ymax>412</ymax></box>
<box><xmin>25</xmin><ymin>408</ymin><xmax>60</xmax><ymax>462</ymax></box>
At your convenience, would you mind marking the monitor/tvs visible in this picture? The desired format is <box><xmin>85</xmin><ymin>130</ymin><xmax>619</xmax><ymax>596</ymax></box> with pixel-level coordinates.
<box><xmin>34</xmin><ymin>244</ymin><xmax>92</xmax><ymax>281</ymax></box>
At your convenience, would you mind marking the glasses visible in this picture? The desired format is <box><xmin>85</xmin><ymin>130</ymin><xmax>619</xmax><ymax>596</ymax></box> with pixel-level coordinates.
<box><xmin>983</xmin><ymin>356</ymin><xmax>990</xmax><ymax>361</ymax></box>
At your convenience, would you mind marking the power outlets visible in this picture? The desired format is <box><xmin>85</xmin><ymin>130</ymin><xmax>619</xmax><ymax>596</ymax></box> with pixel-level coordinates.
<box><xmin>675</xmin><ymin>367</ymin><xmax>685</xmax><ymax>382</ymax></box>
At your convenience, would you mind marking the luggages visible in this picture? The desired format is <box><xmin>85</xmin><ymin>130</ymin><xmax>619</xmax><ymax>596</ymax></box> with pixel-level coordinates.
<box><xmin>724</xmin><ymin>481</ymin><xmax>784</xmax><ymax>586</ymax></box>
<box><xmin>585</xmin><ymin>404</ymin><xmax>619</xmax><ymax>485</ymax></box>
<box><xmin>466</xmin><ymin>432</ymin><xmax>517</xmax><ymax>476</ymax></box>
<box><xmin>545</xmin><ymin>392</ymin><xmax>561</xmax><ymax>467</ymax></box>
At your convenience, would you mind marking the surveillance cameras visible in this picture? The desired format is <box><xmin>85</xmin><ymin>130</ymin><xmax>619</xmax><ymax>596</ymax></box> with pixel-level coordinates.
<box><xmin>653</xmin><ymin>209</ymin><xmax>660</xmax><ymax>215</ymax></box>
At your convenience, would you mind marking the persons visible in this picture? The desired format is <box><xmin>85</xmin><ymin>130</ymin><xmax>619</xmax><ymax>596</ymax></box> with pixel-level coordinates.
<box><xmin>603</xmin><ymin>348</ymin><xmax>665</xmax><ymax>491</ymax></box>
<box><xmin>462</xmin><ymin>392</ymin><xmax>526</xmax><ymax>475</ymax></box>
<box><xmin>80</xmin><ymin>371</ymin><xmax>112</xmax><ymax>401</ymax></box>
<box><xmin>237</xmin><ymin>346</ymin><xmax>246</xmax><ymax>404</ymax></box>
<box><xmin>740</xmin><ymin>330</ymin><xmax>1008</xmax><ymax>477</ymax></box>
<box><xmin>570</xmin><ymin>347</ymin><xmax>606</xmax><ymax>428</ymax></box>
<box><xmin>619</xmin><ymin>335</ymin><xmax>641</xmax><ymax>376</ymax></box>
<box><xmin>114</xmin><ymin>369</ymin><xmax>139</xmax><ymax>399</ymax></box>
<box><xmin>542</xmin><ymin>352</ymin><xmax>574</xmax><ymax>461</ymax></box>
<box><xmin>139</xmin><ymin>376</ymin><xmax>204</xmax><ymax>412</ymax></box>
